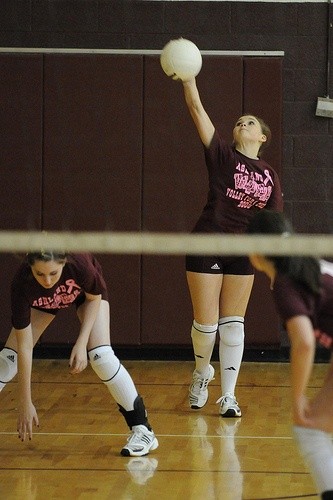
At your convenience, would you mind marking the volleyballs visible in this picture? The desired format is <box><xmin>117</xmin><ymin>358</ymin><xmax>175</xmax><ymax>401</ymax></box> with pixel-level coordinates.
<box><xmin>160</xmin><ymin>39</ymin><xmax>202</xmax><ymax>82</ymax></box>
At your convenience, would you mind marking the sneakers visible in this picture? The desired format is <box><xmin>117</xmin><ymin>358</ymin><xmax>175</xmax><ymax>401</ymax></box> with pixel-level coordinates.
<box><xmin>188</xmin><ymin>366</ymin><xmax>214</xmax><ymax>409</ymax></box>
<box><xmin>219</xmin><ymin>395</ymin><xmax>241</xmax><ymax>417</ymax></box>
<box><xmin>120</xmin><ymin>424</ymin><xmax>159</xmax><ymax>457</ymax></box>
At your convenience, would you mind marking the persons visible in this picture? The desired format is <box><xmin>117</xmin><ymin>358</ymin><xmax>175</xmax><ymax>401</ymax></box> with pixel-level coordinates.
<box><xmin>177</xmin><ymin>38</ymin><xmax>283</xmax><ymax>417</ymax></box>
<box><xmin>247</xmin><ymin>209</ymin><xmax>333</xmax><ymax>500</ymax></box>
<box><xmin>0</xmin><ymin>231</ymin><xmax>158</xmax><ymax>456</ymax></box>
